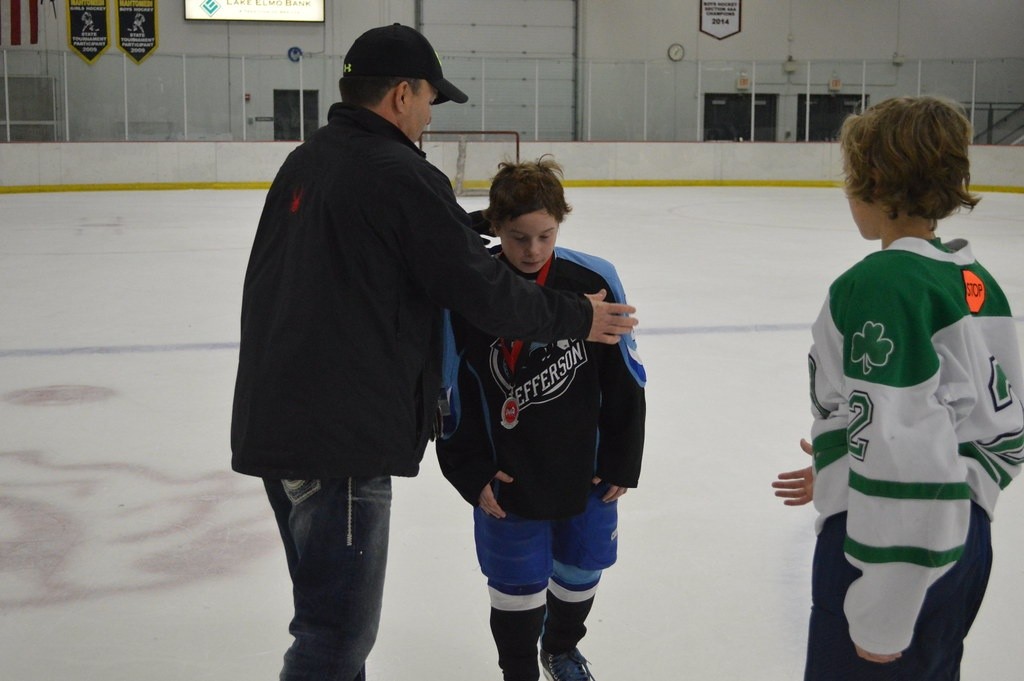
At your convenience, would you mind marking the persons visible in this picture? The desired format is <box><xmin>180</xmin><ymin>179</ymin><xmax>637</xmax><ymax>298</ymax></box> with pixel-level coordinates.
<box><xmin>230</xmin><ymin>23</ymin><xmax>638</xmax><ymax>681</ymax></box>
<box><xmin>436</xmin><ymin>153</ymin><xmax>645</xmax><ymax>680</ymax></box>
<box><xmin>771</xmin><ymin>97</ymin><xmax>1024</xmax><ymax>681</ymax></box>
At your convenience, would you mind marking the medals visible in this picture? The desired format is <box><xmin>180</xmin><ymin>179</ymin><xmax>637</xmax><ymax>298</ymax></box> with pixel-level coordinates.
<box><xmin>500</xmin><ymin>397</ymin><xmax>519</xmax><ymax>430</ymax></box>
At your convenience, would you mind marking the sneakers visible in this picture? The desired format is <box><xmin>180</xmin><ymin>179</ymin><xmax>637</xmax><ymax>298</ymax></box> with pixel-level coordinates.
<box><xmin>539</xmin><ymin>646</ymin><xmax>596</xmax><ymax>681</ymax></box>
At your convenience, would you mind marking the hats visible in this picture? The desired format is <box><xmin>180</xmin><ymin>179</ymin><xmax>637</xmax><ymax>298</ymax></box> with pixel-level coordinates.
<box><xmin>343</xmin><ymin>22</ymin><xmax>469</xmax><ymax>106</ymax></box>
